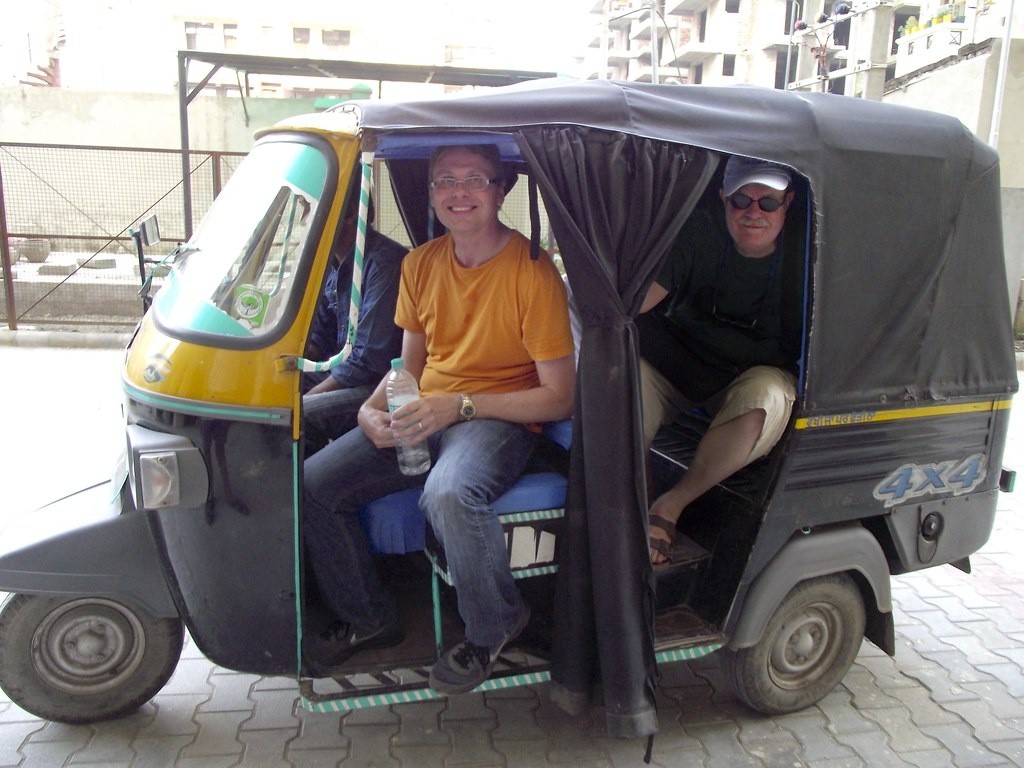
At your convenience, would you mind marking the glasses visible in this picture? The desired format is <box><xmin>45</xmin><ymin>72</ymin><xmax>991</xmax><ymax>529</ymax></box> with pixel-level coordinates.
<box><xmin>727</xmin><ymin>193</ymin><xmax>786</xmax><ymax>212</ymax></box>
<box><xmin>431</xmin><ymin>176</ymin><xmax>504</xmax><ymax>192</ymax></box>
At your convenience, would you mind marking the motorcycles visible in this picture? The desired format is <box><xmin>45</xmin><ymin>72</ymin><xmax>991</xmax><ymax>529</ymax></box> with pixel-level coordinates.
<box><xmin>0</xmin><ymin>78</ymin><xmax>1017</xmax><ymax>727</ymax></box>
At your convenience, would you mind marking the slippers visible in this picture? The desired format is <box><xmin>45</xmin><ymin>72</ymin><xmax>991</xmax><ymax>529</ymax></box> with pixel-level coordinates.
<box><xmin>647</xmin><ymin>514</ymin><xmax>676</xmax><ymax>571</ymax></box>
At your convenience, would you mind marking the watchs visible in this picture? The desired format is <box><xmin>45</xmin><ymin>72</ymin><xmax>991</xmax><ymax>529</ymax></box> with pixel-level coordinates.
<box><xmin>457</xmin><ymin>392</ymin><xmax>476</xmax><ymax>422</ymax></box>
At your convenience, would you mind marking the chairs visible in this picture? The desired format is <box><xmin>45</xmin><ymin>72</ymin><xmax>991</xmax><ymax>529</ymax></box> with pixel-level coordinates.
<box><xmin>361</xmin><ymin>275</ymin><xmax>588</xmax><ymax>558</ymax></box>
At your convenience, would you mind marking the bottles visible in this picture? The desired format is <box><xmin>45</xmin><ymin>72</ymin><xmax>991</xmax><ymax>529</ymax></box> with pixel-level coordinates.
<box><xmin>386</xmin><ymin>357</ymin><xmax>431</xmax><ymax>476</ymax></box>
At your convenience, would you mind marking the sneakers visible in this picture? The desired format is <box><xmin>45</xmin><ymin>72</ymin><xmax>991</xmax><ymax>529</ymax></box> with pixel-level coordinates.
<box><xmin>429</xmin><ymin>598</ymin><xmax>531</xmax><ymax>694</ymax></box>
<box><xmin>302</xmin><ymin>593</ymin><xmax>405</xmax><ymax>666</ymax></box>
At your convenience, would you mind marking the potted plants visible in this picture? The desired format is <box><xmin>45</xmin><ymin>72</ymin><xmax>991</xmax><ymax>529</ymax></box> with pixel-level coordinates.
<box><xmin>896</xmin><ymin>0</ymin><xmax>997</xmax><ymax>40</ymax></box>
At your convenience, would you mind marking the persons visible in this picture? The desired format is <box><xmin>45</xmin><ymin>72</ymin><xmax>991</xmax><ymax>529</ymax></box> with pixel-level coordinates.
<box><xmin>282</xmin><ymin>163</ymin><xmax>405</xmax><ymax>433</ymax></box>
<box><xmin>618</xmin><ymin>151</ymin><xmax>814</xmax><ymax>562</ymax></box>
<box><xmin>291</xmin><ymin>142</ymin><xmax>574</xmax><ymax>697</ymax></box>
